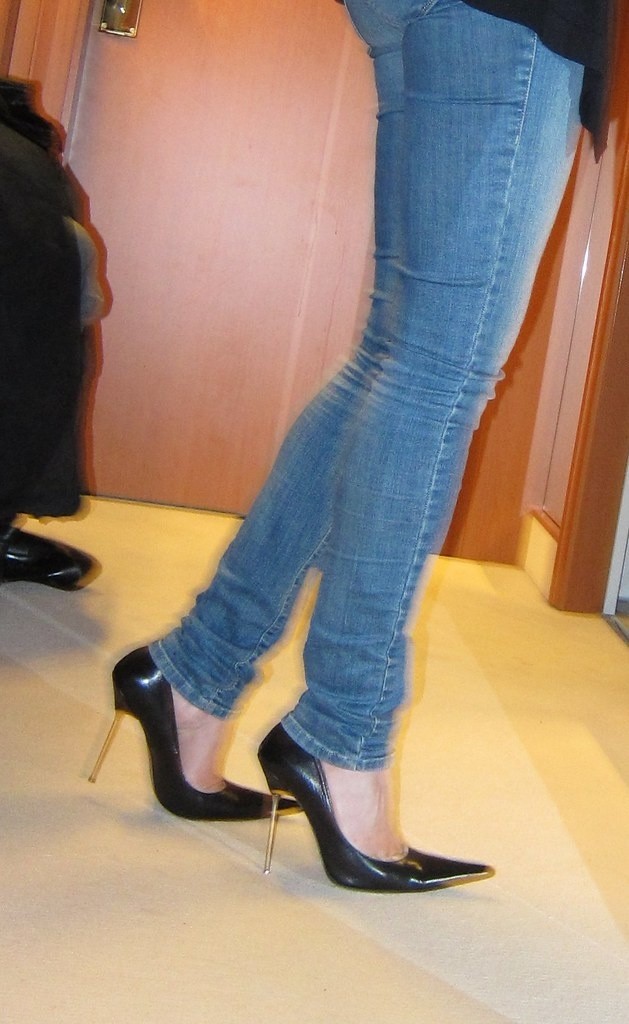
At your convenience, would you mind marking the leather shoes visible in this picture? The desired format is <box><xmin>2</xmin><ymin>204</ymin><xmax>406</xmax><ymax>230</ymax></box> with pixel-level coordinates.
<box><xmin>0</xmin><ymin>526</ymin><xmax>92</xmax><ymax>590</ymax></box>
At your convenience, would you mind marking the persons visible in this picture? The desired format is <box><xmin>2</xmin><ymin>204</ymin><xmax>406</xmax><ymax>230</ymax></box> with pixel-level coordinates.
<box><xmin>0</xmin><ymin>124</ymin><xmax>105</xmax><ymax>589</ymax></box>
<box><xmin>88</xmin><ymin>0</ymin><xmax>629</xmax><ymax>892</ymax></box>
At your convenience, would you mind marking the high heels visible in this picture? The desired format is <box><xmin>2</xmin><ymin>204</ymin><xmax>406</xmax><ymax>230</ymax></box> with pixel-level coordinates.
<box><xmin>88</xmin><ymin>647</ymin><xmax>305</xmax><ymax>821</ymax></box>
<box><xmin>258</xmin><ymin>722</ymin><xmax>495</xmax><ymax>894</ymax></box>
